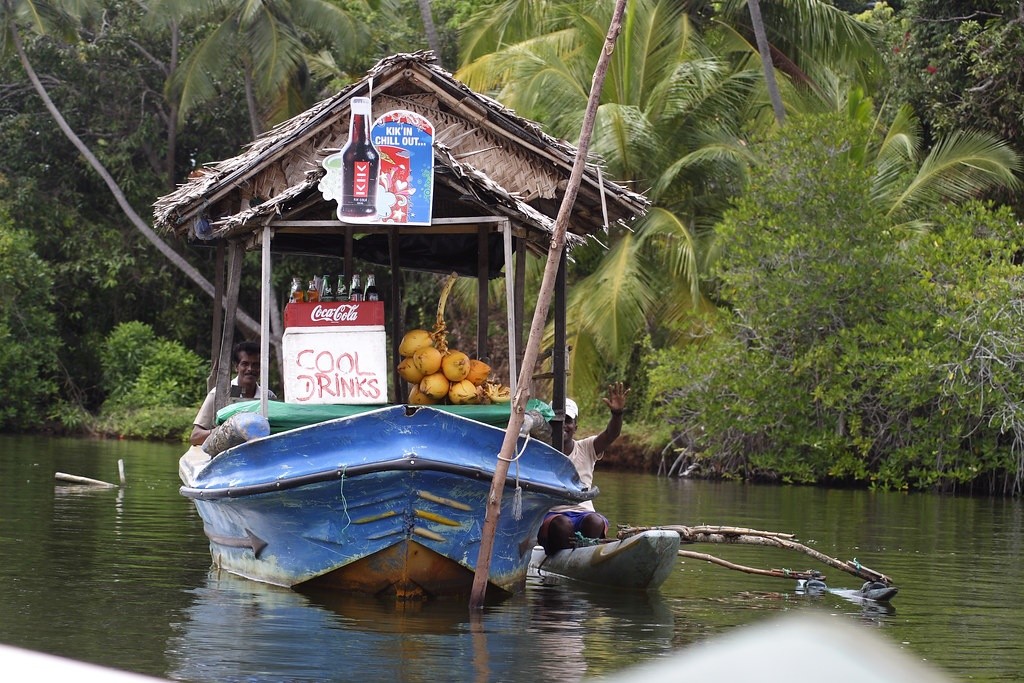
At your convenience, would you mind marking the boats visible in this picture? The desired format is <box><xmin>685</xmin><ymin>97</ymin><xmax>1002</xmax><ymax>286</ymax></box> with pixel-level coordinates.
<box><xmin>148</xmin><ymin>48</ymin><xmax>600</xmax><ymax>597</ymax></box>
<box><xmin>524</xmin><ymin>526</ymin><xmax>681</xmax><ymax>599</ymax></box>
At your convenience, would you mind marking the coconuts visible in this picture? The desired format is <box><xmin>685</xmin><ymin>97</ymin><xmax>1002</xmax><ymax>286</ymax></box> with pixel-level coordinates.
<box><xmin>397</xmin><ymin>330</ymin><xmax>493</xmax><ymax>405</ymax></box>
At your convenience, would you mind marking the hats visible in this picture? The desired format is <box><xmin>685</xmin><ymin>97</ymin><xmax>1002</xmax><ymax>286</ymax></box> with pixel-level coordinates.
<box><xmin>548</xmin><ymin>398</ymin><xmax>578</xmax><ymax>419</ymax></box>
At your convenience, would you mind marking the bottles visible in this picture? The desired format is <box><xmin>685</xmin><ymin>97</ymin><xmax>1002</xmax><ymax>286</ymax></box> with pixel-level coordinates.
<box><xmin>289</xmin><ymin>273</ymin><xmax>379</xmax><ymax>304</ymax></box>
<box><xmin>339</xmin><ymin>96</ymin><xmax>381</xmax><ymax>222</ymax></box>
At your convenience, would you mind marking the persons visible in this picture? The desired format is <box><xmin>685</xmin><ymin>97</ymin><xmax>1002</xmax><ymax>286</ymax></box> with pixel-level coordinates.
<box><xmin>537</xmin><ymin>382</ymin><xmax>630</xmax><ymax>553</ymax></box>
<box><xmin>190</xmin><ymin>341</ymin><xmax>276</xmax><ymax>446</ymax></box>
